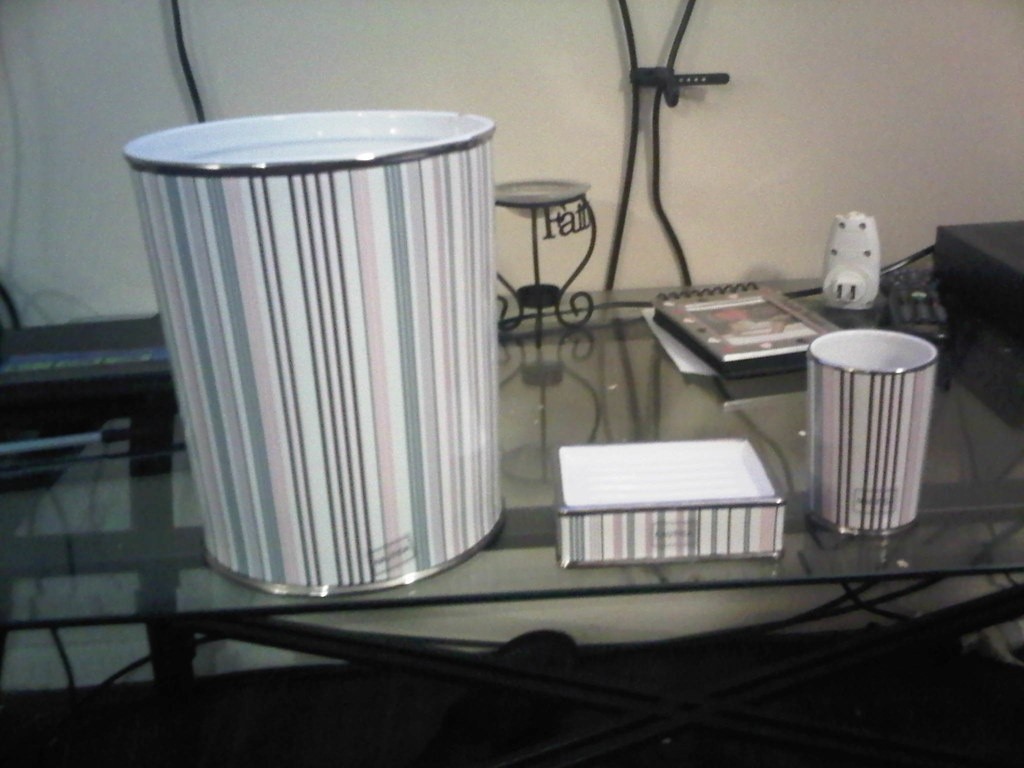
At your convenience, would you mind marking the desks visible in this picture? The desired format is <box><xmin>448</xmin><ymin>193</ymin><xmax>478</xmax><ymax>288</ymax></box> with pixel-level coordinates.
<box><xmin>1</xmin><ymin>274</ymin><xmax>1023</xmax><ymax>768</ymax></box>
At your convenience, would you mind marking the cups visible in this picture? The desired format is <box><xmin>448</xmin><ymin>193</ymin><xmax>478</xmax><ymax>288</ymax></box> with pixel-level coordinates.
<box><xmin>807</xmin><ymin>330</ymin><xmax>939</xmax><ymax>535</ymax></box>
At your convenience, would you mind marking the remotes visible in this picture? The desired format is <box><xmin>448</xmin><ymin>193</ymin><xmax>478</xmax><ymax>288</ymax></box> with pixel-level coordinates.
<box><xmin>889</xmin><ymin>268</ymin><xmax>949</xmax><ymax>340</ymax></box>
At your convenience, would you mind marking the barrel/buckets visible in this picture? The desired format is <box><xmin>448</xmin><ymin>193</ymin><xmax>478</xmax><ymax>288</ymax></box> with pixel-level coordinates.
<box><xmin>122</xmin><ymin>111</ymin><xmax>500</xmax><ymax>594</ymax></box>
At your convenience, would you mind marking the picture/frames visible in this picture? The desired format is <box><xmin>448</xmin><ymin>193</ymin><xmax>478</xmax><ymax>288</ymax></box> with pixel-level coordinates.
<box><xmin>652</xmin><ymin>289</ymin><xmax>848</xmax><ymax>376</ymax></box>
